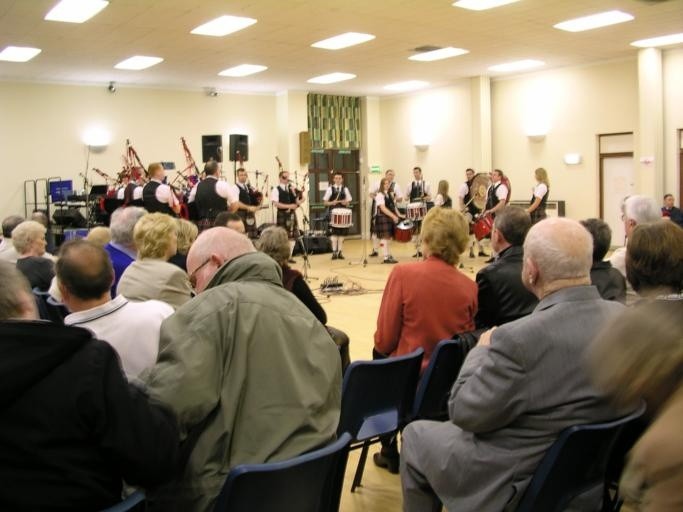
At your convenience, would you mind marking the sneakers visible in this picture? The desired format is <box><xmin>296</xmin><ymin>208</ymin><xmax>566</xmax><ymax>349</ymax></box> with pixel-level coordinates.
<box><xmin>288</xmin><ymin>251</ymin><xmax>490</xmax><ymax>263</ymax></box>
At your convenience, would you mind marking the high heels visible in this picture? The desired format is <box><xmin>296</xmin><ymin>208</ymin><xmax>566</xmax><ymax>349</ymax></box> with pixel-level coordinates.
<box><xmin>373</xmin><ymin>452</ymin><xmax>399</xmax><ymax>473</ymax></box>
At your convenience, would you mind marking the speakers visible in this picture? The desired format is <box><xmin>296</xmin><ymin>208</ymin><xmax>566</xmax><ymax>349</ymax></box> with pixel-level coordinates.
<box><xmin>229</xmin><ymin>134</ymin><xmax>248</xmax><ymax>161</ymax></box>
<box><xmin>202</xmin><ymin>135</ymin><xmax>223</xmax><ymax>162</ymax></box>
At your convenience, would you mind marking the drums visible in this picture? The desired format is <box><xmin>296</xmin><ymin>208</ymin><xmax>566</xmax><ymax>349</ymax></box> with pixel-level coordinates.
<box><xmin>394</xmin><ymin>218</ymin><xmax>415</xmax><ymax>242</ymax></box>
<box><xmin>468</xmin><ymin>173</ymin><xmax>511</xmax><ymax>209</ymax></box>
<box><xmin>472</xmin><ymin>214</ymin><xmax>494</xmax><ymax>241</ymax></box>
<box><xmin>330</xmin><ymin>208</ymin><xmax>353</xmax><ymax>228</ymax></box>
<box><xmin>407</xmin><ymin>201</ymin><xmax>427</xmax><ymax>221</ymax></box>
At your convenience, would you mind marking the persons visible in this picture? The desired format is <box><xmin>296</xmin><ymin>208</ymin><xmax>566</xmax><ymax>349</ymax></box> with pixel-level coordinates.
<box><xmin>1</xmin><ymin>162</ymin><xmax>352</xmax><ymax>471</ymax></box>
<box><xmin>0</xmin><ymin>258</ymin><xmax>178</xmax><ymax>512</ymax></box>
<box><xmin>578</xmin><ymin>221</ymin><xmax>682</xmax><ymax>512</ymax></box>
<box><xmin>369</xmin><ymin>168</ymin><xmax>682</xmax><ymax>330</ymax></box>
<box><xmin>374</xmin><ymin>209</ymin><xmax>481</xmax><ymax>473</ymax></box>
<box><xmin>135</xmin><ymin>226</ymin><xmax>344</xmax><ymax>507</ymax></box>
<box><xmin>401</xmin><ymin>217</ymin><xmax>627</xmax><ymax>511</ymax></box>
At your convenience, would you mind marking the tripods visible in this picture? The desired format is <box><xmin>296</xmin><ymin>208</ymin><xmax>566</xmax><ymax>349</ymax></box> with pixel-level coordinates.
<box><xmin>300</xmin><ymin>226</ymin><xmax>319</xmax><ymax>284</ymax></box>
<box><xmin>348</xmin><ymin>207</ymin><xmax>380</xmax><ymax>267</ymax></box>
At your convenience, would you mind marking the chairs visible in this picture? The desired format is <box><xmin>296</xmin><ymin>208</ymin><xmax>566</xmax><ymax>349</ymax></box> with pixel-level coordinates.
<box><xmin>341</xmin><ymin>349</ymin><xmax>427</xmax><ymax>492</ymax></box>
<box><xmin>419</xmin><ymin>326</ymin><xmax>487</xmax><ymax>411</ymax></box>
<box><xmin>322</xmin><ymin>328</ymin><xmax>352</xmax><ymax>351</ymax></box>
<box><xmin>217</xmin><ymin>433</ymin><xmax>355</xmax><ymax>511</ymax></box>
<box><xmin>96</xmin><ymin>485</ymin><xmax>153</xmax><ymax>512</ymax></box>
<box><xmin>514</xmin><ymin>399</ymin><xmax>650</xmax><ymax>511</ymax></box>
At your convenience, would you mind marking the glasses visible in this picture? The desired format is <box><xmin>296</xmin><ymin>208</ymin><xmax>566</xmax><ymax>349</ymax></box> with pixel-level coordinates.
<box><xmin>186</xmin><ymin>258</ymin><xmax>228</xmax><ymax>289</ymax></box>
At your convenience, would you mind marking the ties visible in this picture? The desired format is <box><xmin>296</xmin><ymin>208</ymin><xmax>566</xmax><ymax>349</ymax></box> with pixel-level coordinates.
<box><xmin>243</xmin><ymin>182</ymin><xmax>419</xmax><ymax>211</ymax></box>
